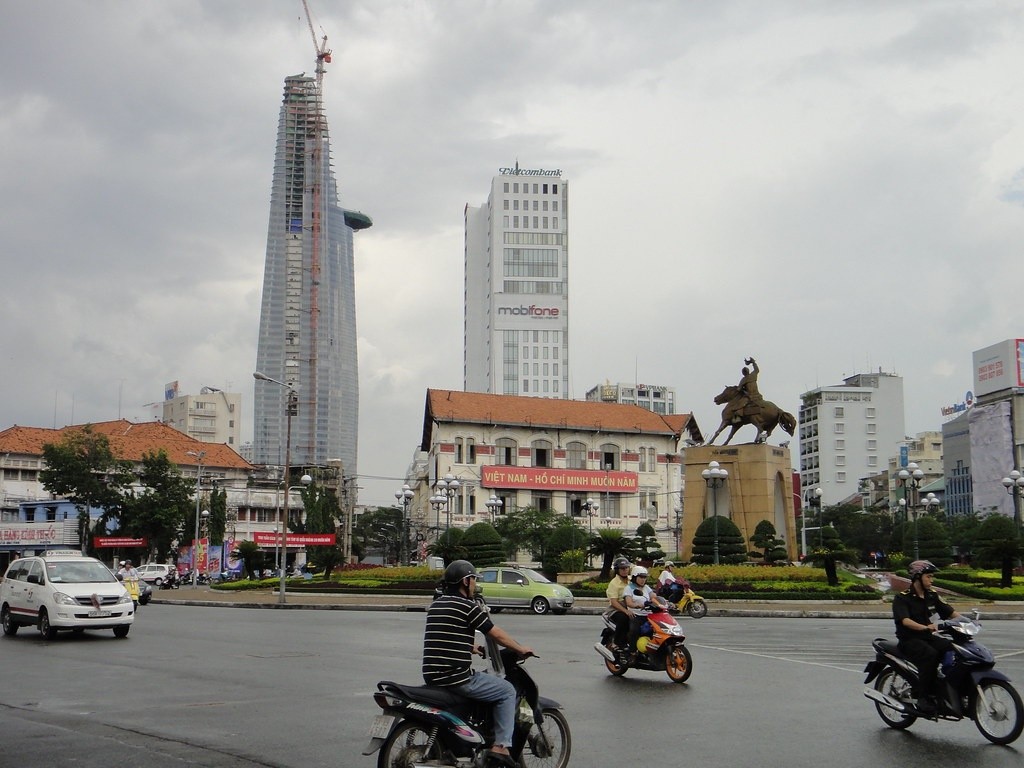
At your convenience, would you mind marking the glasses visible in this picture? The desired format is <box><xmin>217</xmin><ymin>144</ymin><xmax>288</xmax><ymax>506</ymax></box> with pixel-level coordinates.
<box><xmin>470</xmin><ymin>575</ymin><xmax>476</xmax><ymax>580</ymax></box>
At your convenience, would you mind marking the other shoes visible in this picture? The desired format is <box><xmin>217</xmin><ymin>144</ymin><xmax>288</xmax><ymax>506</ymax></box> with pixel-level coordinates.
<box><xmin>918</xmin><ymin>697</ymin><xmax>937</xmax><ymax>711</ymax></box>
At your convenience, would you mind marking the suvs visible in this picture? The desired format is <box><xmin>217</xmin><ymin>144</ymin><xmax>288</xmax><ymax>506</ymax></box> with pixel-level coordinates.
<box><xmin>135</xmin><ymin>564</ymin><xmax>180</xmax><ymax>585</ymax></box>
<box><xmin>466</xmin><ymin>562</ymin><xmax>576</xmax><ymax>614</ymax></box>
<box><xmin>0</xmin><ymin>548</ymin><xmax>134</xmax><ymax>639</ymax></box>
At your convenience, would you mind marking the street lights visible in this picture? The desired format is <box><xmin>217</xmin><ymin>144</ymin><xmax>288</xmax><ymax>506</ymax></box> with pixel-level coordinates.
<box><xmin>816</xmin><ymin>486</ymin><xmax>824</xmax><ymax>550</ymax></box>
<box><xmin>583</xmin><ymin>497</ymin><xmax>600</xmax><ymax>567</ymax></box>
<box><xmin>395</xmin><ymin>473</ymin><xmax>503</xmax><ymax>567</ymax></box>
<box><xmin>253</xmin><ymin>372</ymin><xmax>312</xmax><ymax>603</ymax></box>
<box><xmin>1001</xmin><ymin>469</ymin><xmax>1024</xmax><ymax>540</ymax></box>
<box><xmin>186</xmin><ymin>452</ymin><xmax>210</xmax><ymax>589</ymax></box>
<box><xmin>897</xmin><ymin>461</ymin><xmax>942</xmax><ymax>562</ymax></box>
<box><xmin>701</xmin><ymin>460</ymin><xmax>728</xmax><ymax>565</ymax></box>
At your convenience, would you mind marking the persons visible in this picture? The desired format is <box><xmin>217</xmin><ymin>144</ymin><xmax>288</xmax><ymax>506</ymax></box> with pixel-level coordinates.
<box><xmin>658</xmin><ymin>561</ymin><xmax>683</xmax><ymax>608</ymax></box>
<box><xmin>893</xmin><ymin>560</ymin><xmax>973</xmax><ymax>712</ymax></box>
<box><xmin>731</xmin><ymin>357</ymin><xmax>761</xmax><ymax>422</ymax></box>
<box><xmin>116</xmin><ymin>559</ymin><xmax>233</xmax><ymax>590</ymax></box>
<box><xmin>606</xmin><ymin>557</ymin><xmax>634</xmax><ymax>652</ymax></box>
<box><xmin>254</xmin><ymin>564</ymin><xmax>302</xmax><ymax>579</ymax></box>
<box><xmin>622</xmin><ymin>566</ymin><xmax>670</xmax><ymax>653</ymax></box>
<box><xmin>422</xmin><ymin>560</ymin><xmax>533</xmax><ymax>768</ymax></box>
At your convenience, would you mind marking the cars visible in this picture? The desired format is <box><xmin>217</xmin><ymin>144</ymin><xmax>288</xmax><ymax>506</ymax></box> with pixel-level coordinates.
<box><xmin>132</xmin><ymin>576</ymin><xmax>153</xmax><ymax>606</ymax></box>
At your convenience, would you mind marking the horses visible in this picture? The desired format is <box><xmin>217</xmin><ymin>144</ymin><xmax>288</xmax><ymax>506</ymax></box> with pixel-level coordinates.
<box><xmin>705</xmin><ymin>385</ymin><xmax>797</xmax><ymax>444</ymax></box>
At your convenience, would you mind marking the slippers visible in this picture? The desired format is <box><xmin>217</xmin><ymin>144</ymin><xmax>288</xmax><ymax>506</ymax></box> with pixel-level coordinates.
<box><xmin>486</xmin><ymin>750</ymin><xmax>517</xmax><ymax>768</ymax></box>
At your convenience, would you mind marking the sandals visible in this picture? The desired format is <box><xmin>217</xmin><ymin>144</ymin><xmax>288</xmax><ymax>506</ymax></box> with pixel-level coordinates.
<box><xmin>611</xmin><ymin>644</ymin><xmax>623</xmax><ymax>653</ymax></box>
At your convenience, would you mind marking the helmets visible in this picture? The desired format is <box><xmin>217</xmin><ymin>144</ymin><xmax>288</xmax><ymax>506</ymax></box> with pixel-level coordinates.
<box><xmin>126</xmin><ymin>559</ymin><xmax>132</xmax><ymax>564</ymax></box>
<box><xmin>119</xmin><ymin>561</ymin><xmax>125</xmax><ymax>566</ymax></box>
<box><xmin>908</xmin><ymin>560</ymin><xmax>940</xmax><ymax>581</ymax></box>
<box><xmin>630</xmin><ymin>566</ymin><xmax>648</xmax><ymax>581</ymax></box>
<box><xmin>614</xmin><ymin>557</ymin><xmax>632</xmax><ymax>570</ymax></box>
<box><xmin>170</xmin><ymin>568</ymin><xmax>177</xmax><ymax>572</ymax></box>
<box><xmin>665</xmin><ymin>560</ymin><xmax>674</xmax><ymax>569</ymax></box>
<box><xmin>444</xmin><ymin>559</ymin><xmax>485</xmax><ymax>583</ymax></box>
<box><xmin>636</xmin><ymin>636</ymin><xmax>651</xmax><ymax>653</ymax></box>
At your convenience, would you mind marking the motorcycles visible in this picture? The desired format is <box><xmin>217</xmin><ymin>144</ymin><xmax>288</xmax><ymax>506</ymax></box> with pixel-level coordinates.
<box><xmin>118</xmin><ymin>572</ymin><xmax>144</xmax><ymax>613</ymax></box>
<box><xmin>862</xmin><ymin>607</ymin><xmax>1024</xmax><ymax>745</ymax></box>
<box><xmin>594</xmin><ymin>596</ymin><xmax>693</xmax><ymax>683</ymax></box>
<box><xmin>654</xmin><ymin>581</ymin><xmax>708</xmax><ymax>620</ymax></box>
<box><xmin>158</xmin><ymin>568</ymin><xmax>212</xmax><ymax>589</ymax></box>
<box><xmin>360</xmin><ymin>646</ymin><xmax>573</xmax><ymax>768</ymax></box>
